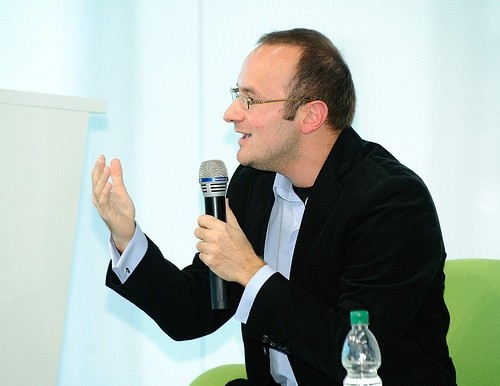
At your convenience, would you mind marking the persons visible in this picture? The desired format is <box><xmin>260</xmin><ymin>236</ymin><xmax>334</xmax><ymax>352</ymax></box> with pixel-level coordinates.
<box><xmin>90</xmin><ymin>27</ymin><xmax>460</xmax><ymax>386</ymax></box>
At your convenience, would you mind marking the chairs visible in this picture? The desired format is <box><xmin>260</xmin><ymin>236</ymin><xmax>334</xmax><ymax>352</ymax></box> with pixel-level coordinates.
<box><xmin>189</xmin><ymin>258</ymin><xmax>500</xmax><ymax>386</ymax></box>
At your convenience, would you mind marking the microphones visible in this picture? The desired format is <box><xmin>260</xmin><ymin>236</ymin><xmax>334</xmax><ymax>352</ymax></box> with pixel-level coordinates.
<box><xmin>198</xmin><ymin>160</ymin><xmax>235</xmax><ymax>315</ymax></box>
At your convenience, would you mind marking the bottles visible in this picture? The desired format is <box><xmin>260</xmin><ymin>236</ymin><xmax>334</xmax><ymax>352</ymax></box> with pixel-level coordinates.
<box><xmin>341</xmin><ymin>311</ymin><xmax>382</xmax><ymax>386</ymax></box>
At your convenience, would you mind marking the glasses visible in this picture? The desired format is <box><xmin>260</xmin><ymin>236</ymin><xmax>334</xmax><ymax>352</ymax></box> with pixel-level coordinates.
<box><xmin>230</xmin><ymin>88</ymin><xmax>314</xmax><ymax>110</ymax></box>
